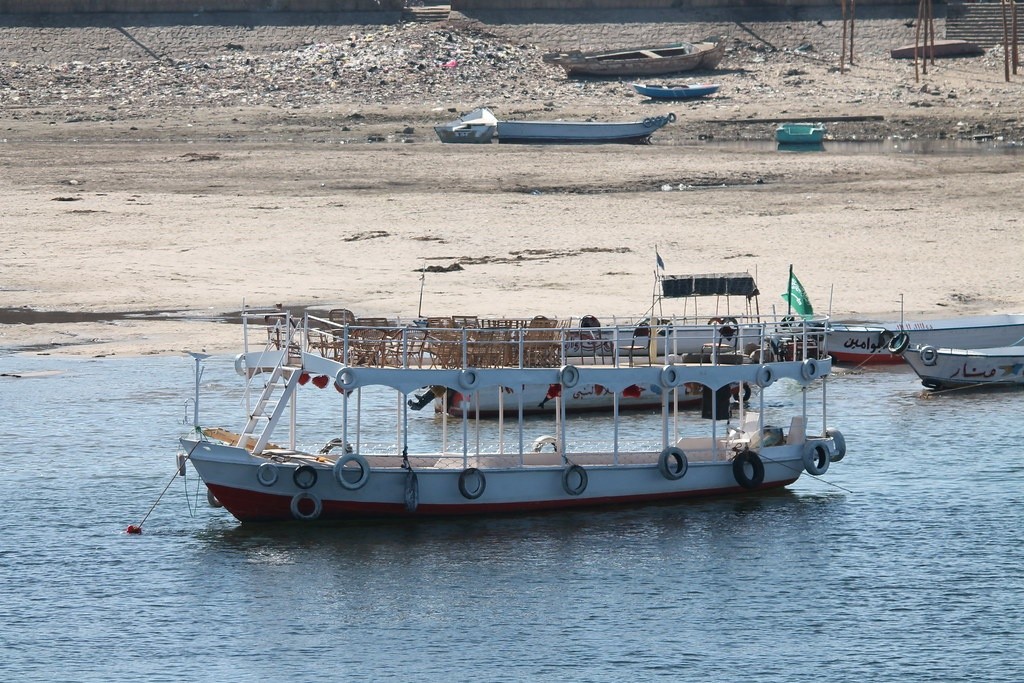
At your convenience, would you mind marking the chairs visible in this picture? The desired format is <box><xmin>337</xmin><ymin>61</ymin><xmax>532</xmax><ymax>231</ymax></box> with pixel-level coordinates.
<box><xmin>721</xmin><ymin>412</ymin><xmax>760</xmax><ymax>459</ymax></box>
<box><xmin>265</xmin><ymin>309</ymin><xmax>660</xmax><ymax>367</ymax></box>
<box><xmin>699</xmin><ymin>317</ymin><xmax>739</xmax><ymax>366</ymax></box>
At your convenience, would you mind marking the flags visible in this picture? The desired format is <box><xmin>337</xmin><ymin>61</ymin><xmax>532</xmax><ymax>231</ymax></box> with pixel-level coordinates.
<box><xmin>781</xmin><ymin>272</ymin><xmax>813</xmax><ymax>321</ymax></box>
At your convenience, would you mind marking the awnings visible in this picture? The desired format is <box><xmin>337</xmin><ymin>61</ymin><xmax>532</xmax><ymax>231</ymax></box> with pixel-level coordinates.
<box><xmin>657</xmin><ymin>253</ymin><xmax>664</xmax><ymax>270</ymax></box>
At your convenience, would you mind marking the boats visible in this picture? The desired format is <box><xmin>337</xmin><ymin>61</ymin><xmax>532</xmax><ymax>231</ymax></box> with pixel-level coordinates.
<box><xmin>635</xmin><ymin>83</ymin><xmax>719</xmax><ymax>100</ymax></box>
<box><xmin>434</xmin><ymin>108</ymin><xmax>671</xmax><ymax>146</ymax></box>
<box><xmin>177</xmin><ymin>315</ymin><xmax>1024</xmax><ymax>527</ymax></box>
<box><xmin>542</xmin><ymin>35</ymin><xmax>728</xmax><ymax>77</ymax></box>
<box><xmin>891</xmin><ymin>39</ymin><xmax>983</xmax><ymax>59</ymax></box>
<box><xmin>895</xmin><ymin>341</ymin><xmax>1024</xmax><ymax>387</ymax></box>
<box><xmin>428</xmin><ymin>272</ymin><xmax>757</xmax><ymax>416</ymax></box>
<box><xmin>775</xmin><ymin>122</ymin><xmax>826</xmax><ymax>145</ymax></box>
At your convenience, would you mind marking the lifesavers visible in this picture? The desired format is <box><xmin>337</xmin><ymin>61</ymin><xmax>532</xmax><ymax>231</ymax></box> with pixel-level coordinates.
<box><xmin>802</xmin><ymin>440</ymin><xmax>830</xmax><ymax>475</ymax></box>
<box><xmin>335</xmin><ymin>366</ymin><xmax>359</xmax><ymax>389</ymax></box>
<box><xmin>562</xmin><ymin>463</ymin><xmax>589</xmax><ymax>495</ymax></box>
<box><xmin>821</xmin><ymin>428</ymin><xmax>846</xmax><ymax>462</ymax></box>
<box><xmin>660</xmin><ymin>365</ymin><xmax>680</xmax><ymax>388</ymax></box>
<box><xmin>889</xmin><ymin>332</ymin><xmax>909</xmax><ymax>354</ymax></box>
<box><xmin>293</xmin><ymin>465</ymin><xmax>319</xmax><ymax>489</ymax></box>
<box><xmin>643</xmin><ymin>111</ymin><xmax>677</xmax><ymax>129</ymax></box>
<box><xmin>256</xmin><ymin>463</ymin><xmax>280</xmax><ymax>487</ymax></box>
<box><xmin>802</xmin><ymin>357</ymin><xmax>820</xmax><ymax>380</ymax></box>
<box><xmin>334</xmin><ymin>454</ymin><xmax>370</xmax><ymax>490</ymax></box>
<box><xmin>658</xmin><ymin>446</ymin><xmax>689</xmax><ymax>480</ymax></box>
<box><xmin>290</xmin><ymin>491</ymin><xmax>323</xmax><ymax>522</ymax></box>
<box><xmin>919</xmin><ymin>344</ymin><xmax>938</xmax><ymax>366</ymax></box>
<box><xmin>458</xmin><ymin>367</ymin><xmax>481</xmax><ymax>389</ymax></box>
<box><xmin>757</xmin><ymin>365</ymin><xmax>775</xmax><ymax>387</ymax></box>
<box><xmin>558</xmin><ymin>364</ymin><xmax>580</xmax><ymax>388</ymax></box>
<box><xmin>733</xmin><ymin>451</ymin><xmax>765</xmax><ymax>489</ymax></box>
<box><xmin>207</xmin><ymin>489</ymin><xmax>223</xmax><ymax>507</ymax></box>
<box><xmin>458</xmin><ymin>467</ymin><xmax>486</xmax><ymax>500</ymax></box>
